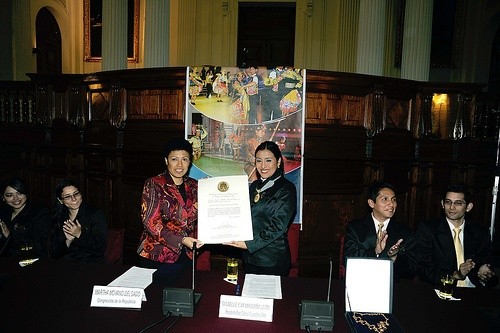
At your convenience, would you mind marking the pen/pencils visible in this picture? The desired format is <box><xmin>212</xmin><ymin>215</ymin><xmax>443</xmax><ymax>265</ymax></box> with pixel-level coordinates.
<box><xmin>236</xmin><ymin>285</ymin><xmax>240</xmax><ymax>295</ymax></box>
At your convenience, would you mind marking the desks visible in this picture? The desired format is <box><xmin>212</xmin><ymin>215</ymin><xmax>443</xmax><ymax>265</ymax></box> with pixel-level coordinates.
<box><xmin>82</xmin><ymin>264</ymin><xmax>500</xmax><ymax>333</ymax></box>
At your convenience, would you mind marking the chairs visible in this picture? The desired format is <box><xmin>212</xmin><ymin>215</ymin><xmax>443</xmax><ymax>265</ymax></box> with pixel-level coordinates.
<box><xmin>101</xmin><ymin>227</ymin><xmax>124</xmax><ymax>277</ymax></box>
<box><xmin>287</xmin><ymin>223</ymin><xmax>300</xmax><ymax>276</ymax></box>
<box><xmin>339</xmin><ymin>233</ymin><xmax>348</xmax><ymax>278</ymax></box>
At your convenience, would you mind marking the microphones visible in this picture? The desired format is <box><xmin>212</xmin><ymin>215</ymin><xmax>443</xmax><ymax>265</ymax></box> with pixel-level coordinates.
<box><xmin>192</xmin><ymin>241</ymin><xmax>196</xmax><ymax>291</ymax></box>
<box><xmin>327</xmin><ymin>256</ymin><xmax>333</xmax><ymax>302</ymax></box>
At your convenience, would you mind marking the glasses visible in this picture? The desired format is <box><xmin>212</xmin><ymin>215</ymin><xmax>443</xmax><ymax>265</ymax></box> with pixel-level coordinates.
<box><xmin>441</xmin><ymin>199</ymin><xmax>466</xmax><ymax>206</ymax></box>
<box><xmin>61</xmin><ymin>192</ymin><xmax>81</xmax><ymax>200</ymax></box>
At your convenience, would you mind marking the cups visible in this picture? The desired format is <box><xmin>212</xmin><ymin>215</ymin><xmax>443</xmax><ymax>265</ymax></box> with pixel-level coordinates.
<box><xmin>226</xmin><ymin>258</ymin><xmax>240</xmax><ymax>281</ymax></box>
<box><xmin>440</xmin><ymin>274</ymin><xmax>455</xmax><ymax>296</ymax></box>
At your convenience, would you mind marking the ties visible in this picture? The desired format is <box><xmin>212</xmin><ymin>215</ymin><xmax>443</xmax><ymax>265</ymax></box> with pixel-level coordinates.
<box><xmin>378</xmin><ymin>225</ymin><xmax>384</xmax><ymax>236</ymax></box>
<box><xmin>453</xmin><ymin>227</ymin><xmax>467</xmax><ymax>287</ymax></box>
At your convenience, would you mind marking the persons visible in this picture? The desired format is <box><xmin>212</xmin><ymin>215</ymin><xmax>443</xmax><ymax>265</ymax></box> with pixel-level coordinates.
<box><xmin>412</xmin><ymin>187</ymin><xmax>500</xmax><ymax>287</ymax></box>
<box><xmin>138</xmin><ymin>141</ymin><xmax>211</xmax><ymax>274</ymax></box>
<box><xmin>0</xmin><ymin>180</ymin><xmax>51</xmax><ymax>274</ymax></box>
<box><xmin>51</xmin><ymin>180</ymin><xmax>110</xmax><ymax>283</ymax></box>
<box><xmin>339</xmin><ymin>182</ymin><xmax>403</xmax><ymax>288</ymax></box>
<box><xmin>222</xmin><ymin>141</ymin><xmax>298</xmax><ymax>279</ymax></box>
<box><xmin>189</xmin><ymin>67</ymin><xmax>302</xmax><ymax>162</ymax></box>
